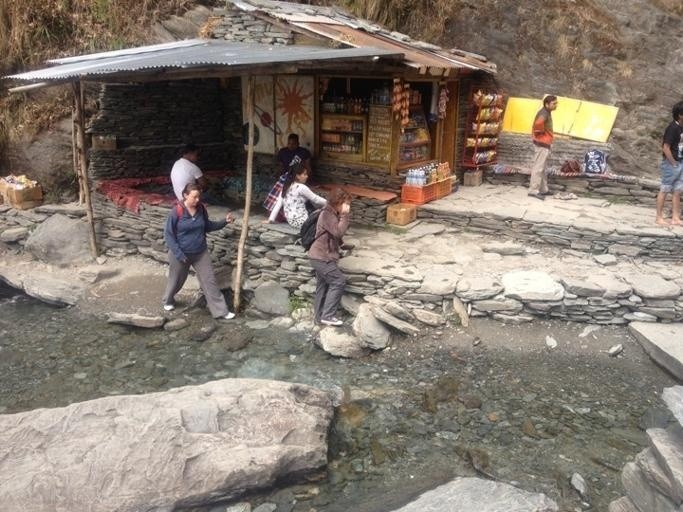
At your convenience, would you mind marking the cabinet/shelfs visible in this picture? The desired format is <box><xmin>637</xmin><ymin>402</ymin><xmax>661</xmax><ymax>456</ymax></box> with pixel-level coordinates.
<box><xmin>398</xmin><ymin>105</ymin><xmax>432</xmax><ymax>166</ymax></box>
<box><xmin>462</xmin><ymin>84</ymin><xmax>503</xmax><ymax>168</ymax></box>
<box><xmin>321</xmin><ymin>113</ymin><xmax>367</xmax><ymax>163</ymax></box>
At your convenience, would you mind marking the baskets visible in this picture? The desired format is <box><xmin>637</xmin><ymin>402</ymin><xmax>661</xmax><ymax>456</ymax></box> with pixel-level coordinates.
<box><xmin>401</xmin><ymin>178</ymin><xmax>452</xmax><ymax>204</ymax></box>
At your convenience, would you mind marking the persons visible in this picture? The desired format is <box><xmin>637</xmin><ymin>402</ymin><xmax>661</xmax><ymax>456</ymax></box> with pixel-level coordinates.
<box><xmin>170</xmin><ymin>142</ymin><xmax>224</xmax><ymax>204</ymax></box>
<box><xmin>307</xmin><ymin>186</ymin><xmax>353</xmax><ymax>328</ymax></box>
<box><xmin>526</xmin><ymin>96</ymin><xmax>558</xmax><ymax>200</ymax></box>
<box><xmin>281</xmin><ymin>163</ymin><xmax>355</xmax><ymax>260</ymax></box>
<box><xmin>654</xmin><ymin>101</ymin><xmax>683</xmax><ymax>228</ymax></box>
<box><xmin>161</xmin><ymin>183</ymin><xmax>238</xmax><ymax>321</ymax></box>
<box><xmin>274</xmin><ymin>132</ymin><xmax>314</xmax><ymax>186</ymax></box>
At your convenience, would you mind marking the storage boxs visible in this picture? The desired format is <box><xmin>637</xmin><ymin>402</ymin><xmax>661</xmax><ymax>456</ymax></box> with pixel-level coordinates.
<box><xmin>0</xmin><ymin>178</ymin><xmax>43</xmax><ymax>211</ymax></box>
<box><xmin>386</xmin><ymin>203</ymin><xmax>416</xmax><ymax>225</ymax></box>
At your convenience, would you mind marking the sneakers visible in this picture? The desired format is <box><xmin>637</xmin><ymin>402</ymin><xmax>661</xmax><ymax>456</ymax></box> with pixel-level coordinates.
<box><xmin>221</xmin><ymin>312</ymin><xmax>235</xmax><ymax>320</ymax></box>
<box><xmin>314</xmin><ymin>317</ymin><xmax>343</xmax><ymax>327</ymax></box>
<box><xmin>528</xmin><ymin>191</ymin><xmax>553</xmax><ymax>200</ymax></box>
<box><xmin>342</xmin><ymin>244</ymin><xmax>354</xmax><ymax>250</ymax></box>
<box><xmin>654</xmin><ymin>218</ymin><xmax>683</xmax><ymax>228</ymax></box>
<box><xmin>163</xmin><ymin>304</ymin><xmax>175</xmax><ymax>311</ymax></box>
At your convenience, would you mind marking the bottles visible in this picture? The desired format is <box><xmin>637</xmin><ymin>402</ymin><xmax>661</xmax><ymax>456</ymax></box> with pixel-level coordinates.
<box><xmin>406</xmin><ymin>162</ymin><xmax>450</xmax><ymax>186</ymax></box>
<box><xmin>320</xmin><ymin>88</ymin><xmax>392</xmax><ymax>160</ymax></box>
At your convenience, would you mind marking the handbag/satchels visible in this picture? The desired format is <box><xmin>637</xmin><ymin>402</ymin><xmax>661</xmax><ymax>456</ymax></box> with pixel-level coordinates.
<box><xmin>560</xmin><ymin>160</ymin><xmax>580</xmax><ymax>173</ymax></box>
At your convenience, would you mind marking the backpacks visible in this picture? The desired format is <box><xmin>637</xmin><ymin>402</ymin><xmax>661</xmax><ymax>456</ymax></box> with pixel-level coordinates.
<box><xmin>584</xmin><ymin>150</ymin><xmax>606</xmax><ymax>173</ymax></box>
<box><xmin>300</xmin><ymin>209</ymin><xmax>328</xmax><ymax>251</ymax></box>
